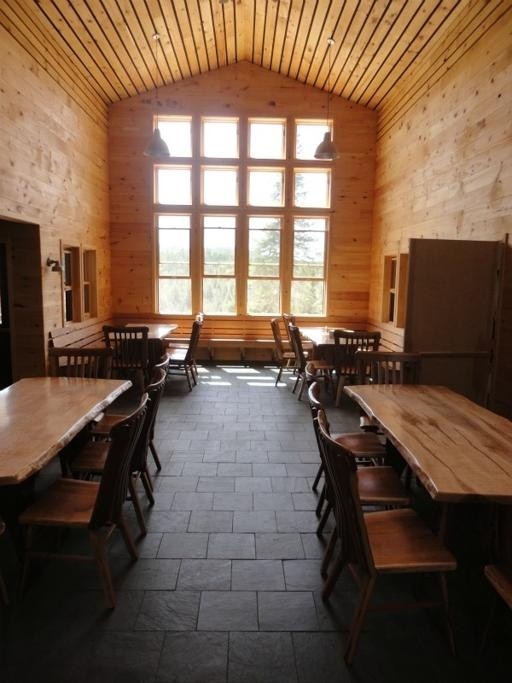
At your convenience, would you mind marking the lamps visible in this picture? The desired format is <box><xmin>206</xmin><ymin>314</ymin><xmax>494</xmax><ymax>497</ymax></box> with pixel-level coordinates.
<box><xmin>316</xmin><ymin>38</ymin><xmax>342</xmax><ymax>159</ymax></box>
<box><xmin>45</xmin><ymin>257</ymin><xmax>63</xmax><ymax>272</ymax></box>
<box><xmin>142</xmin><ymin>32</ymin><xmax>170</xmax><ymax>158</ymax></box>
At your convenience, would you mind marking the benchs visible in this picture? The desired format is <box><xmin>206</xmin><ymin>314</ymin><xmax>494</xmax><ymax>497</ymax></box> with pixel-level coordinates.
<box><xmin>164</xmin><ymin>336</ymin><xmax>313</xmax><ymax>363</ymax></box>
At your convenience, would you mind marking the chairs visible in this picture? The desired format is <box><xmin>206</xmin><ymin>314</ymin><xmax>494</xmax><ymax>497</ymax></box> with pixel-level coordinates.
<box><xmin>13</xmin><ymin>390</ymin><xmax>150</xmax><ymax>611</ymax></box>
<box><xmin>314</xmin><ymin>408</ymin><xmax>412</xmax><ymax>578</ymax></box>
<box><xmin>319</xmin><ymin>425</ymin><xmax>457</xmax><ymax>664</ymax></box>
<box><xmin>87</xmin><ymin>307</ymin><xmax>206</xmax><ymax>395</ymax></box>
<box><xmin>65</xmin><ymin>368</ymin><xmax>166</xmax><ymax>537</ymax></box>
<box><xmin>88</xmin><ymin>350</ymin><xmax>172</xmax><ymax>493</ymax></box>
<box><xmin>307</xmin><ymin>380</ymin><xmax>387</xmax><ymax>517</ymax></box>
<box><xmin>459</xmin><ymin>559</ymin><xmax>512</xmax><ymax>683</ymax></box>
<box><xmin>268</xmin><ymin>313</ymin><xmax>381</xmax><ymax>406</ymax></box>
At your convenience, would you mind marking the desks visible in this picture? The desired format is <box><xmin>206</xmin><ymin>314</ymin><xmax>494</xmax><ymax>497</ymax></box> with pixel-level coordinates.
<box><xmin>0</xmin><ymin>375</ymin><xmax>133</xmax><ymax>575</ymax></box>
<box><xmin>341</xmin><ymin>381</ymin><xmax>511</xmax><ymax>607</ymax></box>
